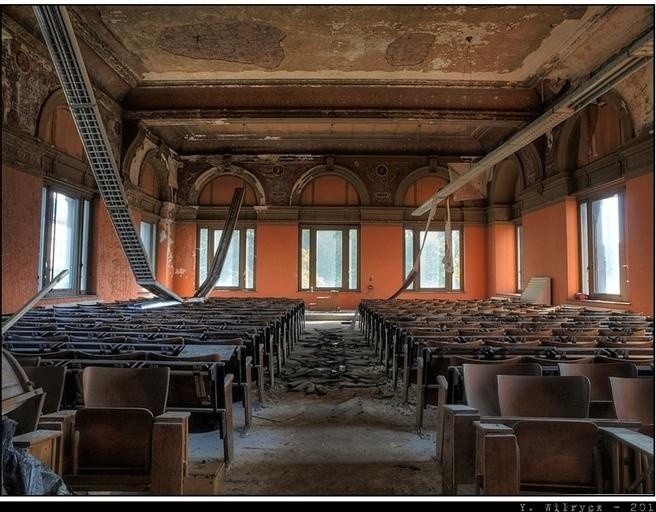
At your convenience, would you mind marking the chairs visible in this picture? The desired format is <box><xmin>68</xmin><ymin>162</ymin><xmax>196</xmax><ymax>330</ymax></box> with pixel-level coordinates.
<box><xmin>1</xmin><ymin>295</ymin><xmax>655</xmax><ymax>497</ymax></box>
